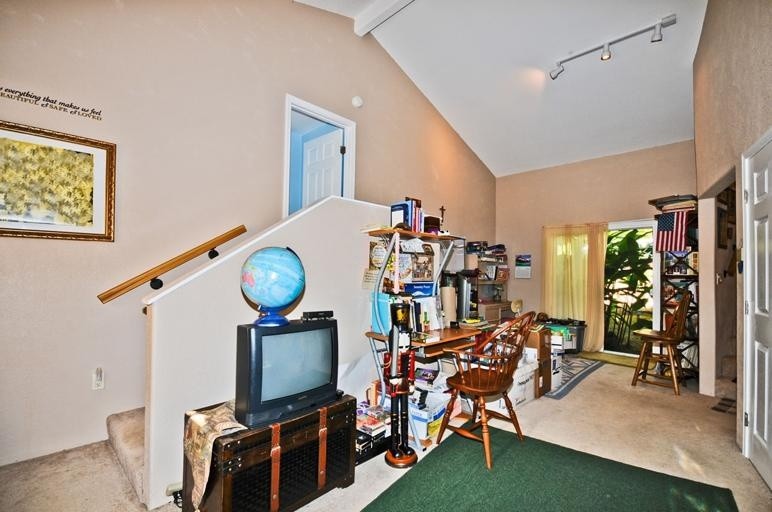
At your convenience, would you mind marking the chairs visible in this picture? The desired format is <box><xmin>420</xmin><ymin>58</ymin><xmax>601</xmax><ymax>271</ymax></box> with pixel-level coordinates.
<box><xmin>435</xmin><ymin>310</ymin><xmax>536</xmax><ymax>471</ymax></box>
<box><xmin>631</xmin><ymin>289</ymin><xmax>693</xmax><ymax>396</ymax></box>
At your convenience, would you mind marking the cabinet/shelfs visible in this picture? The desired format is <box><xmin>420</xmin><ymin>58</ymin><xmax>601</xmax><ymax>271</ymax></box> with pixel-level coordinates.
<box><xmin>647</xmin><ymin>194</ymin><xmax>699</xmax><ymax>384</ymax></box>
<box><xmin>464</xmin><ymin>250</ymin><xmax>519</xmax><ymax>325</ymax></box>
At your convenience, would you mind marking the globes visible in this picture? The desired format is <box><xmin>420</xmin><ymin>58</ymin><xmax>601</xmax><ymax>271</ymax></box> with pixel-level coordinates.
<box><xmin>240</xmin><ymin>246</ymin><xmax>305</xmax><ymax>327</ymax></box>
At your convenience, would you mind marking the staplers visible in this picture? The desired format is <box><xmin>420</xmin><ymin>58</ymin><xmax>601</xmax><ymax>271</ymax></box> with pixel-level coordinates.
<box><xmin>450</xmin><ymin>322</ymin><xmax>460</xmax><ymax>329</ymax></box>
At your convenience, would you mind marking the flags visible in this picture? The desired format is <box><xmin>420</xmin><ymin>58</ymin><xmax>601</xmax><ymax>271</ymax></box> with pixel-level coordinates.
<box><xmin>656</xmin><ymin>210</ymin><xmax>685</xmax><ymax>251</ymax></box>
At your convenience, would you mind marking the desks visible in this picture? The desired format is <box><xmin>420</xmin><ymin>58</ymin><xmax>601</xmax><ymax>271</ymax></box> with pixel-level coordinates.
<box><xmin>363</xmin><ymin>225</ymin><xmax>485</xmax><ymax>457</ymax></box>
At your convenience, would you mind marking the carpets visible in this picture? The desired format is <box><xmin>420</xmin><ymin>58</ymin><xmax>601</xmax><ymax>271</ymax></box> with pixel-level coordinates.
<box><xmin>359</xmin><ymin>418</ymin><xmax>738</xmax><ymax>511</ymax></box>
<box><xmin>543</xmin><ymin>357</ymin><xmax>607</xmax><ymax>400</ymax></box>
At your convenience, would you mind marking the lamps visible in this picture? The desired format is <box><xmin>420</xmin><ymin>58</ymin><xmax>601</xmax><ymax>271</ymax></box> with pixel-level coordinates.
<box><xmin>548</xmin><ymin>15</ymin><xmax>678</xmax><ymax>81</ymax></box>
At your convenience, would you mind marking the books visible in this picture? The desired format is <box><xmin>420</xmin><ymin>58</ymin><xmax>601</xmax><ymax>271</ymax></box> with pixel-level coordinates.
<box><xmin>457</xmin><ymin>274</ymin><xmax>472</xmax><ymax>319</ymax></box>
<box><xmin>370</xmin><ymin>291</ymin><xmax>443</xmax><ymax>343</ymax></box>
<box><xmin>355</xmin><ymin>367</ymin><xmax>456</xmax><ymax>455</ymax></box>
<box><xmin>391</xmin><ymin>197</ymin><xmax>441</xmax><ymax>234</ymax></box>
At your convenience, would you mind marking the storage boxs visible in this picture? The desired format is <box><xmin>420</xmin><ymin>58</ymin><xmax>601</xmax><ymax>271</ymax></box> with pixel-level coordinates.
<box><xmin>181</xmin><ymin>392</ymin><xmax>357</xmax><ymax>511</ymax></box>
<box><xmin>380</xmin><ymin>321</ymin><xmax>592</xmax><ymax>442</ymax></box>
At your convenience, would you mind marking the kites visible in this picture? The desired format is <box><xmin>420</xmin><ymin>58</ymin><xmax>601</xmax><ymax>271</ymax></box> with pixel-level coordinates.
<box><xmin>466</xmin><ymin>239</ymin><xmax>508</xmax><ymax>263</ymax></box>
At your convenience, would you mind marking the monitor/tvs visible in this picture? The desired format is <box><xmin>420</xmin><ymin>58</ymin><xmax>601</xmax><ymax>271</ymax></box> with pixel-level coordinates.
<box><xmin>235</xmin><ymin>318</ymin><xmax>344</xmax><ymax>431</ymax></box>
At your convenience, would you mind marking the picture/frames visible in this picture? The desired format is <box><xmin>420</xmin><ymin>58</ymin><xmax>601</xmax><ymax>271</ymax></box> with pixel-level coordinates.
<box><xmin>0</xmin><ymin>119</ymin><xmax>118</xmax><ymax>244</ymax></box>
